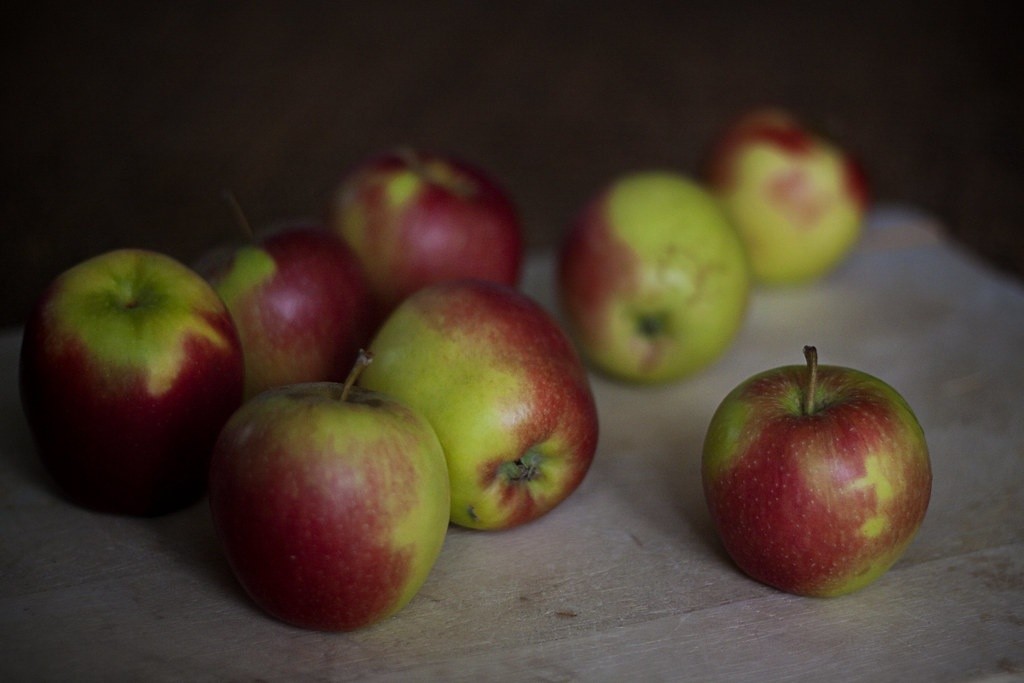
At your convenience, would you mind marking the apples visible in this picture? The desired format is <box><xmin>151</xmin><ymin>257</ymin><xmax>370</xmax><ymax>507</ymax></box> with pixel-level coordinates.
<box><xmin>19</xmin><ymin>246</ymin><xmax>241</xmax><ymax>513</ymax></box>
<box><xmin>191</xmin><ymin>230</ymin><xmax>375</xmax><ymax>401</ymax></box>
<box><xmin>356</xmin><ymin>279</ymin><xmax>600</xmax><ymax>532</ymax></box>
<box><xmin>205</xmin><ymin>350</ymin><xmax>450</xmax><ymax>632</ymax></box>
<box><xmin>560</xmin><ymin>173</ymin><xmax>750</xmax><ymax>385</ymax></box>
<box><xmin>332</xmin><ymin>146</ymin><xmax>524</xmax><ymax>329</ymax></box>
<box><xmin>699</xmin><ymin>344</ymin><xmax>934</xmax><ymax>600</ymax></box>
<box><xmin>697</xmin><ymin>113</ymin><xmax>868</xmax><ymax>286</ymax></box>
